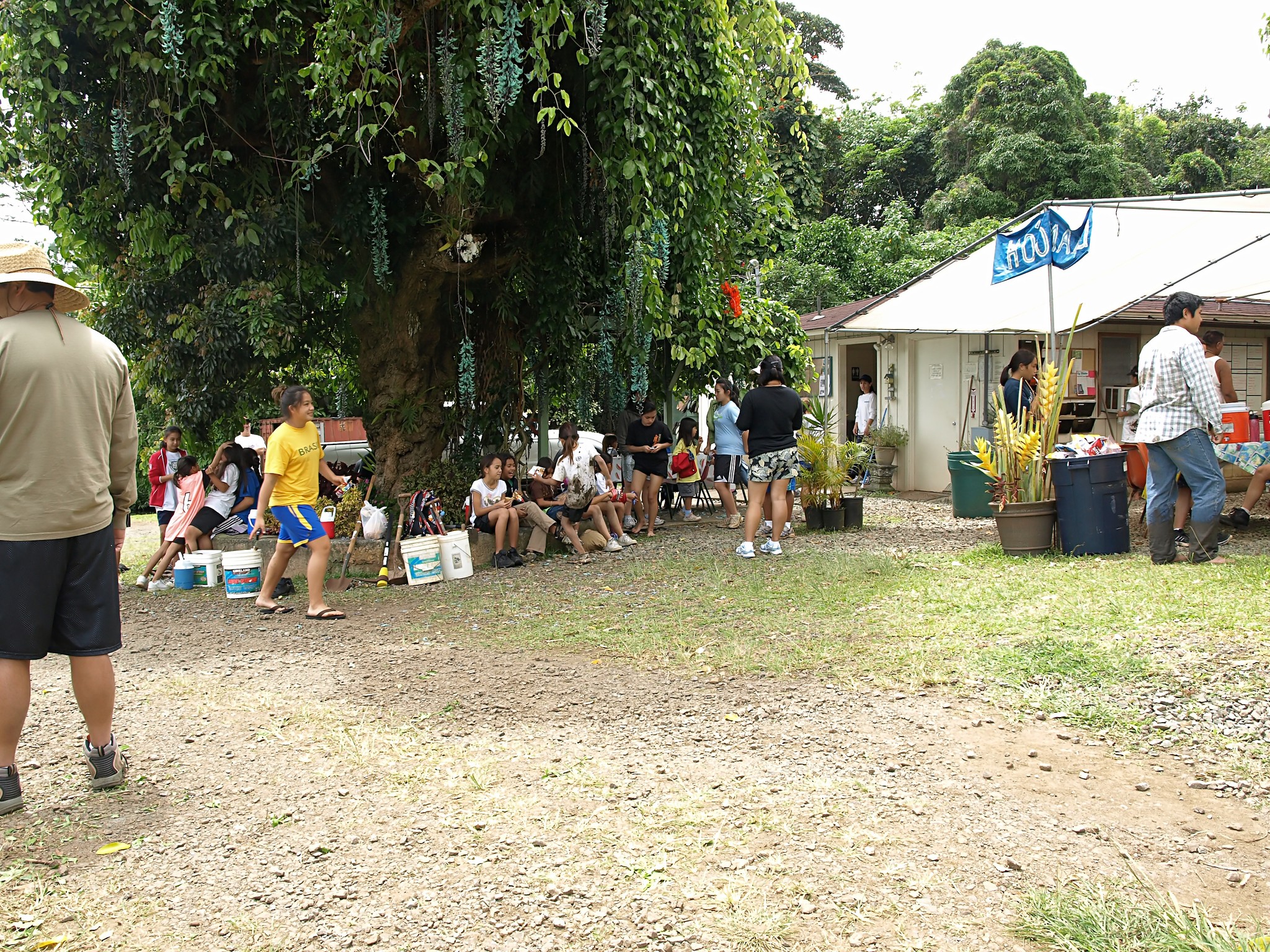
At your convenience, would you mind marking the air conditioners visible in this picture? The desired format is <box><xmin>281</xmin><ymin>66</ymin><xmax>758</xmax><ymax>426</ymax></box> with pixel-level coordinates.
<box><xmin>1100</xmin><ymin>386</ymin><xmax>1132</xmax><ymax>412</ymax></box>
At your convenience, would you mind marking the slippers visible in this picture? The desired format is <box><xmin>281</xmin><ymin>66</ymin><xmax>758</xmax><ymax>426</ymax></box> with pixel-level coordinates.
<box><xmin>259</xmin><ymin>605</ymin><xmax>293</xmax><ymax>613</ymax></box>
<box><xmin>305</xmin><ymin>605</ymin><xmax>345</xmax><ymax>620</ymax></box>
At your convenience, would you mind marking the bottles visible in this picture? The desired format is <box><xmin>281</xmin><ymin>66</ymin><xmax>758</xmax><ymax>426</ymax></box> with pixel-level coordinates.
<box><xmin>1117</xmin><ymin>405</ymin><xmax>1125</xmax><ymax>424</ymax></box>
<box><xmin>1249</xmin><ymin>410</ymin><xmax>1260</xmax><ymax>442</ymax></box>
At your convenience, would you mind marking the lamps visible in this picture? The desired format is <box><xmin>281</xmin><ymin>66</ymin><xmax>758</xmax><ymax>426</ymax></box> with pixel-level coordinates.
<box><xmin>873</xmin><ymin>334</ymin><xmax>895</xmax><ymax>351</ymax></box>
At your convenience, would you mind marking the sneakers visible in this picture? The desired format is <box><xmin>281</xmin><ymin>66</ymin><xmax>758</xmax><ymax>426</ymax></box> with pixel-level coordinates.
<box><xmin>779</xmin><ymin>526</ymin><xmax>795</xmax><ymax>539</ymax></box>
<box><xmin>83</xmin><ymin>730</ymin><xmax>128</xmax><ymax>789</ymax></box>
<box><xmin>117</xmin><ymin>564</ymin><xmax>176</xmax><ymax>592</ymax></box>
<box><xmin>507</xmin><ymin>547</ymin><xmax>523</xmax><ymax>565</ymax></box>
<box><xmin>604</xmin><ymin>509</ymin><xmax>743</xmax><ymax>552</ymax></box>
<box><xmin>519</xmin><ymin>551</ymin><xmax>537</xmax><ymax>563</ymax></box>
<box><xmin>755</xmin><ymin>523</ymin><xmax>774</xmax><ymax>537</ymax></box>
<box><xmin>567</xmin><ymin>552</ymin><xmax>591</xmax><ymax>564</ymax></box>
<box><xmin>759</xmin><ymin>538</ymin><xmax>782</xmax><ymax>555</ymax></box>
<box><xmin>554</xmin><ymin>526</ymin><xmax>567</xmax><ymax>540</ymax></box>
<box><xmin>556</xmin><ymin>512</ymin><xmax>578</xmax><ymax>525</ymax></box>
<box><xmin>1173</xmin><ymin>529</ymin><xmax>1190</xmax><ymax>547</ymax></box>
<box><xmin>736</xmin><ymin>542</ymin><xmax>756</xmax><ymax>558</ymax></box>
<box><xmin>491</xmin><ymin>550</ymin><xmax>515</xmax><ymax>568</ymax></box>
<box><xmin>849</xmin><ymin>475</ymin><xmax>863</xmax><ymax>484</ymax></box>
<box><xmin>860</xmin><ymin>477</ymin><xmax>871</xmax><ymax>484</ymax></box>
<box><xmin>562</xmin><ymin>530</ymin><xmax>584</xmax><ymax>545</ymax></box>
<box><xmin>1217</xmin><ymin>533</ymin><xmax>1233</xmax><ymax>546</ymax></box>
<box><xmin>0</xmin><ymin>765</ymin><xmax>23</xmax><ymax>815</ymax></box>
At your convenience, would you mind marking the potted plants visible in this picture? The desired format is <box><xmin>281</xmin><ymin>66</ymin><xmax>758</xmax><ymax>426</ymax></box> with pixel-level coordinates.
<box><xmin>863</xmin><ymin>416</ymin><xmax>909</xmax><ymax>465</ymax></box>
<box><xmin>796</xmin><ymin>423</ymin><xmax>865</xmax><ymax>531</ymax></box>
<box><xmin>959</xmin><ymin>340</ymin><xmax>1075</xmax><ymax>556</ymax></box>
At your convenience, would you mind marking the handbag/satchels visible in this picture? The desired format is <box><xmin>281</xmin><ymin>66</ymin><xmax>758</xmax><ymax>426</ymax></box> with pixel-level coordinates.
<box><xmin>360</xmin><ymin>499</ymin><xmax>389</xmax><ymax>541</ymax></box>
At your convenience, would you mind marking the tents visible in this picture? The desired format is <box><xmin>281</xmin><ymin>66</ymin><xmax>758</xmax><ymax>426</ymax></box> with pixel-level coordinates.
<box><xmin>823</xmin><ymin>187</ymin><xmax>1270</xmax><ymax>501</ymax></box>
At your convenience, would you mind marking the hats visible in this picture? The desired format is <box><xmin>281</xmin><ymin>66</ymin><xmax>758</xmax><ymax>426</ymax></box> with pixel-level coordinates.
<box><xmin>0</xmin><ymin>244</ymin><xmax>90</xmax><ymax>313</ymax></box>
<box><xmin>858</xmin><ymin>374</ymin><xmax>871</xmax><ymax>384</ymax></box>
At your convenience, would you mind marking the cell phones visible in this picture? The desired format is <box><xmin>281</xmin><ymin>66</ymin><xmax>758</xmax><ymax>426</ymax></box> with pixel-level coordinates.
<box><xmin>650</xmin><ymin>447</ymin><xmax>655</xmax><ymax>450</ymax></box>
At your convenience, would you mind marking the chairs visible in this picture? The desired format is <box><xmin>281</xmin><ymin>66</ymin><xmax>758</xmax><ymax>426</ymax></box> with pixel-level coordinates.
<box><xmin>677</xmin><ymin>464</ymin><xmax>716</xmax><ymax>516</ymax></box>
<box><xmin>1120</xmin><ymin>444</ymin><xmax>1147</xmax><ymax>523</ymax></box>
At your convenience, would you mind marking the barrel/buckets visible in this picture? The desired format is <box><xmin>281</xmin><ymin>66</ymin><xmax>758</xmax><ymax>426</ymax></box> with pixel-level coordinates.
<box><xmin>437</xmin><ymin>530</ymin><xmax>473</xmax><ymax>580</ymax></box>
<box><xmin>173</xmin><ymin>560</ymin><xmax>194</xmax><ymax>590</ymax></box>
<box><xmin>399</xmin><ymin>535</ymin><xmax>444</xmax><ymax>586</ymax></box>
<box><xmin>248</xmin><ymin>509</ymin><xmax>261</xmax><ymax>535</ymax></box>
<box><xmin>947</xmin><ymin>450</ymin><xmax>996</xmax><ymax>519</ymax></box>
<box><xmin>221</xmin><ymin>548</ymin><xmax>262</xmax><ymax>599</ymax></box>
<box><xmin>183</xmin><ymin>550</ymin><xmax>225</xmax><ymax>587</ymax></box>
<box><xmin>1220</xmin><ymin>401</ymin><xmax>1249</xmax><ymax>443</ymax></box>
<box><xmin>320</xmin><ymin>506</ymin><xmax>336</xmax><ymax>539</ymax></box>
<box><xmin>1260</xmin><ymin>400</ymin><xmax>1270</xmax><ymax>441</ymax></box>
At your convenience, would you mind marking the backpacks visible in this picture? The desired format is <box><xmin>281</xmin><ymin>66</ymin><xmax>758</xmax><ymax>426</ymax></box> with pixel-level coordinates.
<box><xmin>403</xmin><ymin>489</ymin><xmax>445</xmax><ymax>537</ymax></box>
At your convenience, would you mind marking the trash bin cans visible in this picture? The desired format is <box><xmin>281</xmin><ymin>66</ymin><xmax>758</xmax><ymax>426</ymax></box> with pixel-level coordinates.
<box><xmin>947</xmin><ymin>450</ymin><xmax>995</xmax><ymax>517</ymax></box>
<box><xmin>1046</xmin><ymin>450</ymin><xmax>1130</xmax><ymax>557</ymax></box>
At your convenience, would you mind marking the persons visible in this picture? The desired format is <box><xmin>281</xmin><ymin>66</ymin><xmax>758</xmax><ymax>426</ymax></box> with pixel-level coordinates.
<box><xmin>1116</xmin><ymin>292</ymin><xmax>1269</xmax><ymax>564</ymax></box>
<box><xmin>0</xmin><ymin>242</ymin><xmax>139</xmax><ymax>814</ymax></box>
<box><xmin>247</xmin><ymin>385</ymin><xmax>346</xmax><ymax>620</ymax></box>
<box><xmin>463</xmin><ymin>355</ymin><xmax>824</xmax><ymax>568</ymax></box>
<box><xmin>848</xmin><ymin>374</ymin><xmax>877</xmax><ymax>483</ymax></box>
<box><xmin>999</xmin><ymin>350</ymin><xmax>1039</xmax><ymax>436</ymax></box>
<box><xmin>116</xmin><ymin>417</ymin><xmax>267</xmax><ymax>592</ymax></box>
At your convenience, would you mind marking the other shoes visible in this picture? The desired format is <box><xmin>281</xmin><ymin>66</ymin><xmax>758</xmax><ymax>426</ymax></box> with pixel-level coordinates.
<box><xmin>1219</xmin><ymin>507</ymin><xmax>1249</xmax><ymax>531</ymax></box>
<box><xmin>1170</xmin><ymin>553</ymin><xmax>1187</xmax><ymax>563</ymax></box>
<box><xmin>272</xmin><ymin>577</ymin><xmax>295</xmax><ymax>597</ymax></box>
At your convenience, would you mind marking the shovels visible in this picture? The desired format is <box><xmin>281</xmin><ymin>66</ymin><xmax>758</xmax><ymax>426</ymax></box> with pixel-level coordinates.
<box><xmin>322</xmin><ymin>475</ymin><xmax>376</xmax><ymax>593</ymax></box>
<box><xmin>388</xmin><ymin>492</ymin><xmax>412</xmax><ymax>581</ymax></box>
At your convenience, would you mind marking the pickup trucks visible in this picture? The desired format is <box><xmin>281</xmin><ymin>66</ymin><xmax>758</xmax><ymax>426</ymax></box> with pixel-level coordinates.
<box><xmin>318</xmin><ymin>400</ymin><xmax>619</xmax><ymax>486</ymax></box>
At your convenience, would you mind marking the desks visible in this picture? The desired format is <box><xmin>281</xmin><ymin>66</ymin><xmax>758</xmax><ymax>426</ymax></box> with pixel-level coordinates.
<box><xmin>609</xmin><ymin>452</ymin><xmax>714</xmax><ymax>486</ymax></box>
<box><xmin>1143</xmin><ymin>441</ymin><xmax>1270</xmax><ymax>537</ymax></box>
